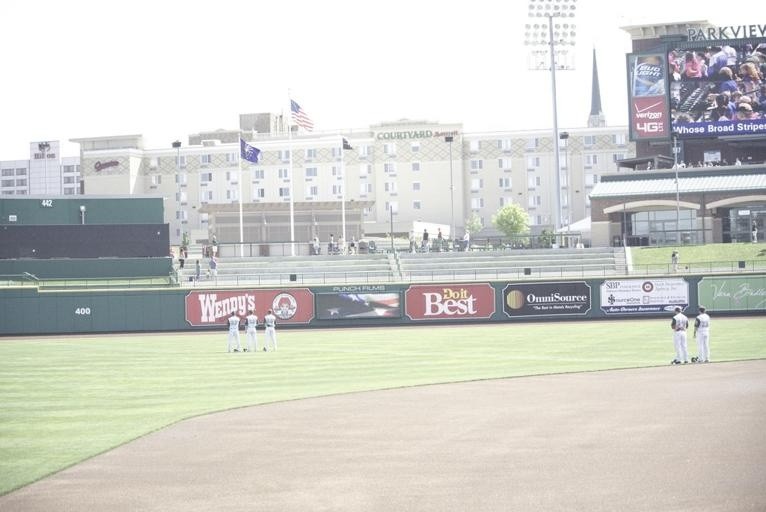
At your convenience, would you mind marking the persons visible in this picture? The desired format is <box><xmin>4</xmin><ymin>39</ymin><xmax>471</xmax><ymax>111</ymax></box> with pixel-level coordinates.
<box><xmin>463</xmin><ymin>229</ymin><xmax>470</xmax><ymax>252</ymax></box>
<box><xmin>409</xmin><ymin>229</ymin><xmax>416</xmax><ymax>254</ymax></box>
<box><xmin>692</xmin><ymin>306</ymin><xmax>713</xmax><ymax>364</ymax></box>
<box><xmin>225</xmin><ymin>310</ymin><xmax>243</xmax><ymax>353</ymax></box>
<box><xmin>750</xmin><ymin>219</ymin><xmax>759</xmax><ymax>242</ymax></box>
<box><xmin>262</xmin><ymin>308</ymin><xmax>278</xmax><ymax>352</ymax></box>
<box><xmin>311</xmin><ymin>233</ymin><xmax>359</xmax><ymax>257</ymax></box>
<box><xmin>436</xmin><ymin>227</ymin><xmax>445</xmax><ymax>252</ymax></box>
<box><xmin>671</xmin><ymin>252</ymin><xmax>679</xmax><ymax>273</ymax></box>
<box><xmin>670</xmin><ymin>306</ymin><xmax>690</xmax><ymax>366</ymax></box>
<box><xmin>421</xmin><ymin>228</ymin><xmax>430</xmax><ymax>253</ymax></box>
<box><xmin>668</xmin><ymin>44</ymin><xmax>766</xmax><ymax>124</ymax></box>
<box><xmin>177</xmin><ymin>234</ymin><xmax>220</xmax><ymax>282</ymax></box>
<box><xmin>243</xmin><ymin>309</ymin><xmax>259</xmax><ymax>353</ymax></box>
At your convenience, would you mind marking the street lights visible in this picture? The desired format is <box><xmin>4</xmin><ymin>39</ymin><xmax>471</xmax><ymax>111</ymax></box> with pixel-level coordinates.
<box><xmin>170</xmin><ymin>140</ymin><xmax>184</xmax><ymax>251</ymax></box>
<box><xmin>38</xmin><ymin>143</ymin><xmax>50</xmax><ymax>195</ymax></box>
<box><xmin>446</xmin><ymin>136</ymin><xmax>453</xmax><ymax>237</ymax></box>
<box><xmin>527</xmin><ymin>0</ymin><xmax>576</xmax><ymax>248</ymax></box>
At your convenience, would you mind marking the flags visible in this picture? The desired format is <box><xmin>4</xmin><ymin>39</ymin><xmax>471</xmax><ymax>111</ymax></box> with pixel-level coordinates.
<box><xmin>239</xmin><ymin>138</ymin><xmax>263</xmax><ymax>168</ymax></box>
<box><xmin>290</xmin><ymin>98</ymin><xmax>316</xmax><ymax>135</ymax></box>
<box><xmin>340</xmin><ymin>135</ymin><xmax>356</xmax><ymax>151</ymax></box>
